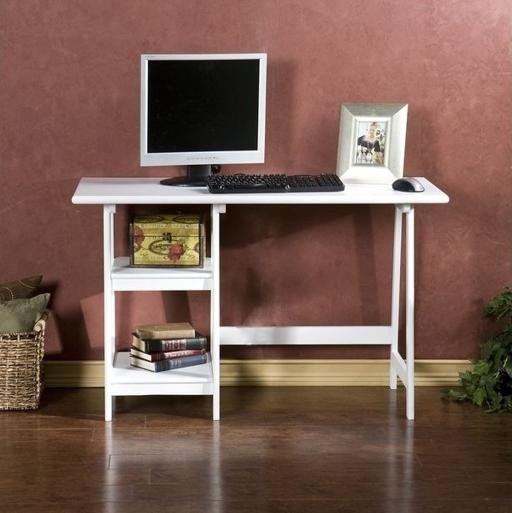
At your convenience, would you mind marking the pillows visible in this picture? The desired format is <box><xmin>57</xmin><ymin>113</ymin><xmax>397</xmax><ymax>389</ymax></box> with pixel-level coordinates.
<box><xmin>0</xmin><ymin>273</ymin><xmax>51</xmax><ymax>334</ymax></box>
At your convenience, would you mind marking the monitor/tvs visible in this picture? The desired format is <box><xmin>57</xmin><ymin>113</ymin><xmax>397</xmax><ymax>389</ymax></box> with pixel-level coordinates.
<box><xmin>140</xmin><ymin>52</ymin><xmax>267</xmax><ymax>186</ymax></box>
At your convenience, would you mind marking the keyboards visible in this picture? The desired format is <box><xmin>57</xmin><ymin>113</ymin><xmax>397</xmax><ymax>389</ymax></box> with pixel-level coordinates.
<box><xmin>206</xmin><ymin>174</ymin><xmax>345</xmax><ymax>193</ymax></box>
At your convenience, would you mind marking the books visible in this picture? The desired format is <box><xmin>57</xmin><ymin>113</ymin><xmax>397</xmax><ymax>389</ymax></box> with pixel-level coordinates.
<box><xmin>131</xmin><ymin>331</ymin><xmax>208</xmax><ymax>353</ymax></box>
<box><xmin>135</xmin><ymin>321</ymin><xmax>196</xmax><ymax>340</ymax></box>
<box><xmin>130</xmin><ymin>344</ymin><xmax>208</xmax><ymax>362</ymax></box>
<box><xmin>129</xmin><ymin>354</ymin><xmax>208</xmax><ymax>372</ymax></box>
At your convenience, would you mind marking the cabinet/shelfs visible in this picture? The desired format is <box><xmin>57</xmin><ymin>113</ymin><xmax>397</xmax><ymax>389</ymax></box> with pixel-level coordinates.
<box><xmin>99</xmin><ymin>205</ymin><xmax>227</xmax><ymax>422</ymax></box>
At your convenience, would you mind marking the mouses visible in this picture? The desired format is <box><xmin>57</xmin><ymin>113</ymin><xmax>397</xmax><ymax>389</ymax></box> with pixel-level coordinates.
<box><xmin>392</xmin><ymin>177</ymin><xmax>425</xmax><ymax>191</ymax></box>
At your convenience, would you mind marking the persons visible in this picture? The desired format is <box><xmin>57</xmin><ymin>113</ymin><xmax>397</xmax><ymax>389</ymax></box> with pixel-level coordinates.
<box><xmin>364</xmin><ymin>122</ymin><xmax>379</xmax><ymax>142</ymax></box>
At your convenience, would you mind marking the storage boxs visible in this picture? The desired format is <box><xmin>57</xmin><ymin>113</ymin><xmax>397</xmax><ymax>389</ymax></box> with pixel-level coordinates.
<box><xmin>127</xmin><ymin>211</ymin><xmax>208</xmax><ymax>270</ymax></box>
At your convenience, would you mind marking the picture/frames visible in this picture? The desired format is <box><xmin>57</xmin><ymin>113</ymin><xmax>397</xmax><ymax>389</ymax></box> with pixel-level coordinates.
<box><xmin>333</xmin><ymin>100</ymin><xmax>410</xmax><ymax>186</ymax></box>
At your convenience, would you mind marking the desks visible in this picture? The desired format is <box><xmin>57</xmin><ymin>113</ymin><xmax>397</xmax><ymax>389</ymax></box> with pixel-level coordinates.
<box><xmin>69</xmin><ymin>177</ymin><xmax>453</xmax><ymax>422</ymax></box>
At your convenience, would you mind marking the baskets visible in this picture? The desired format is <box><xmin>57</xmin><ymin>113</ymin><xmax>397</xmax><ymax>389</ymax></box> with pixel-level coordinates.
<box><xmin>0</xmin><ymin>309</ymin><xmax>51</xmax><ymax>411</ymax></box>
